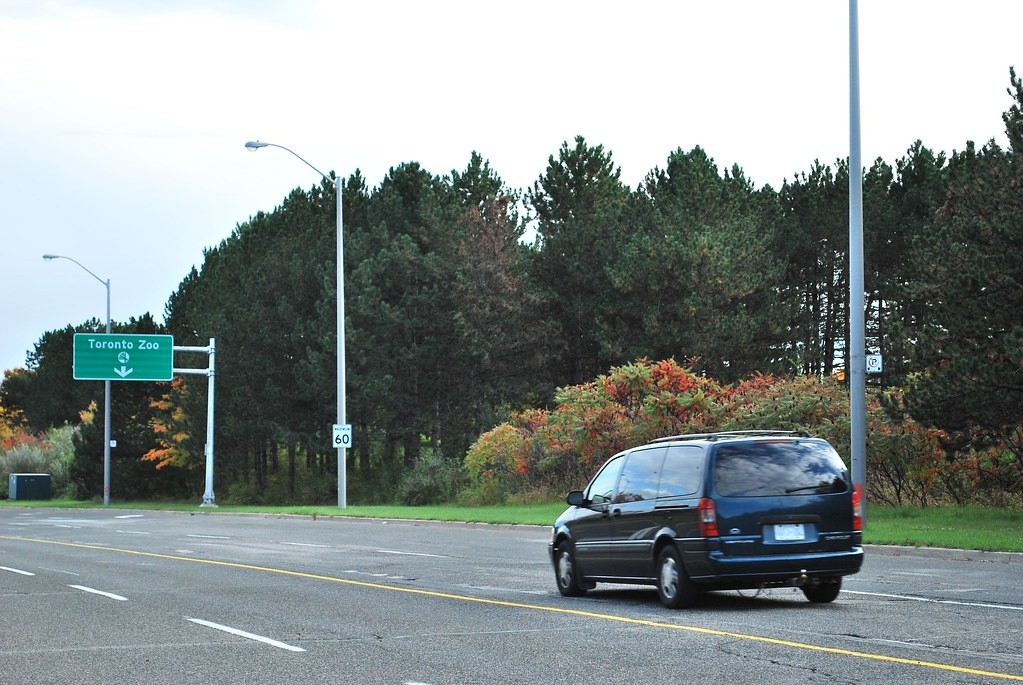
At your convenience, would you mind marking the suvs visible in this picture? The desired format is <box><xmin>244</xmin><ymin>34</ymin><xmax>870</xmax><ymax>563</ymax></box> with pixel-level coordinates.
<box><xmin>547</xmin><ymin>428</ymin><xmax>864</xmax><ymax>609</ymax></box>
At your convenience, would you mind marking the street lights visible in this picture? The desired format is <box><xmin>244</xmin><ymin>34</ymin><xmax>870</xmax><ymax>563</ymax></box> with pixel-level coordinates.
<box><xmin>42</xmin><ymin>253</ymin><xmax>113</xmax><ymax>508</ymax></box>
<box><xmin>242</xmin><ymin>134</ymin><xmax>347</xmax><ymax>511</ymax></box>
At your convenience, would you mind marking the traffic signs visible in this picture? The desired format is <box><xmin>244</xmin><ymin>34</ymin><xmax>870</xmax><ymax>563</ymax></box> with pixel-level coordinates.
<box><xmin>73</xmin><ymin>332</ymin><xmax>173</xmax><ymax>381</ymax></box>
